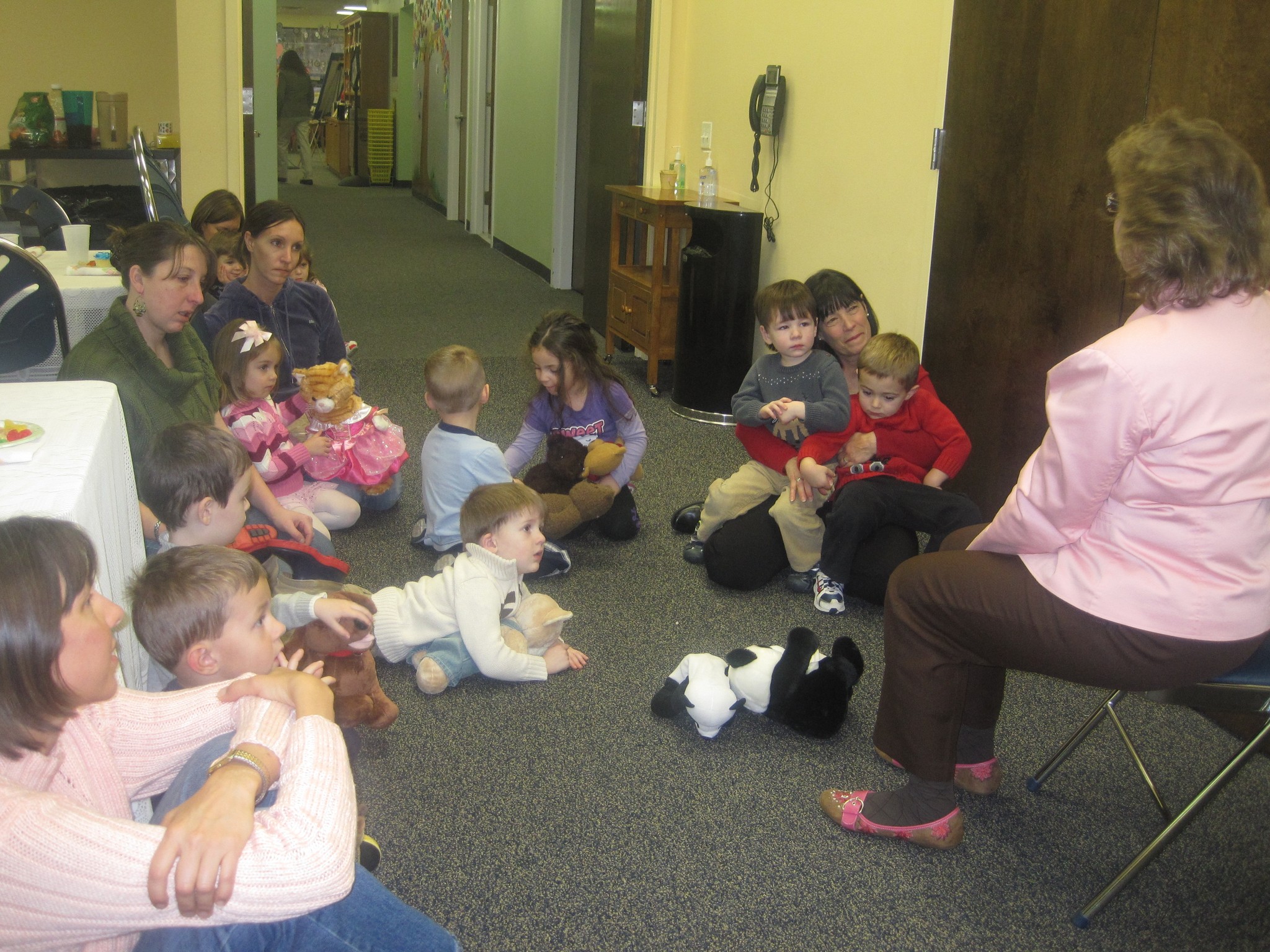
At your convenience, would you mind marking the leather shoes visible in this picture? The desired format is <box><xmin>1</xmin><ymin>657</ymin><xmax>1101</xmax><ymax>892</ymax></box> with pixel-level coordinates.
<box><xmin>671</xmin><ymin>500</ymin><xmax>705</xmax><ymax>533</ymax></box>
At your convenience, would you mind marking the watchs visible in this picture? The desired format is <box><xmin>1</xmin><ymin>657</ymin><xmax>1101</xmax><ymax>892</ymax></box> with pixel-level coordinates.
<box><xmin>208</xmin><ymin>747</ymin><xmax>270</xmax><ymax>806</ymax></box>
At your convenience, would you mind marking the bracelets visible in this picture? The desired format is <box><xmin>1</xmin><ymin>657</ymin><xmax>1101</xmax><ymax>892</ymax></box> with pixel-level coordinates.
<box><xmin>154</xmin><ymin>520</ymin><xmax>163</xmax><ymax>543</ymax></box>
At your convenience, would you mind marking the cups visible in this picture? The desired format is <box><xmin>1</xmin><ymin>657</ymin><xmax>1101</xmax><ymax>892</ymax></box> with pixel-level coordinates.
<box><xmin>660</xmin><ymin>172</ymin><xmax>677</xmax><ymax>189</ymax></box>
<box><xmin>61</xmin><ymin>224</ymin><xmax>91</xmax><ymax>263</ymax></box>
<box><xmin>0</xmin><ymin>233</ymin><xmax>19</xmax><ymax>245</ymax></box>
<box><xmin>61</xmin><ymin>90</ymin><xmax>94</xmax><ymax>149</ymax></box>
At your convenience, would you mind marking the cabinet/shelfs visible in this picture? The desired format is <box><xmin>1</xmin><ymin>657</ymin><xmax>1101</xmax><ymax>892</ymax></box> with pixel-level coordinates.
<box><xmin>339</xmin><ymin>10</ymin><xmax>390</xmax><ymax>187</ymax></box>
<box><xmin>324</xmin><ymin>116</ymin><xmax>350</xmax><ymax>179</ymax></box>
<box><xmin>604</xmin><ymin>184</ymin><xmax>740</xmax><ymax>398</ymax></box>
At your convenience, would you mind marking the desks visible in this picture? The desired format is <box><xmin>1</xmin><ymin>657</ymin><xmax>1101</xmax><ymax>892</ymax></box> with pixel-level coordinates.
<box><xmin>0</xmin><ymin>143</ymin><xmax>183</xmax><ymax>206</ymax></box>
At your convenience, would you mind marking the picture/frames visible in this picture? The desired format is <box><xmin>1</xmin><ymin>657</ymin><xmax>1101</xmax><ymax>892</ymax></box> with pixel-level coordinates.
<box><xmin>313</xmin><ymin>52</ymin><xmax>345</xmax><ymax>120</ymax></box>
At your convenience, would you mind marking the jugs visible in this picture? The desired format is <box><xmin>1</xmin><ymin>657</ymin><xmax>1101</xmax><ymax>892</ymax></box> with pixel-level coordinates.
<box><xmin>95</xmin><ymin>91</ymin><xmax>128</xmax><ymax>150</ymax></box>
<box><xmin>49</xmin><ymin>84</ymin><xmax>68</xmax><ymax>148</ymax></box>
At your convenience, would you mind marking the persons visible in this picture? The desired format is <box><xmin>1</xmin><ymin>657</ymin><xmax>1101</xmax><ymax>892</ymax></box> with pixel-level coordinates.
<box><xmin>276</xmin><ymin>50</ymin><xmax>315</xmax><ymax>185</ymax></box>
<box><xmin>0</xmin><ymin>517</ymin><xmax>465</xmax><ymax>952</ymax></box>
<box><xmin>128</xmin><ymin>543</ymin><xmax>380</xmax><ymax>872</ymax></box>
<box><xmin>408</xmin><ymin>345</ymin><xmax>573</xmax><ymax>584</ymax></box>
<box><xmin>139</xmin><ymin>422</ymin><xmax>375</xmax><ymax>692</ymax></box>
<box><xmin>681</xmin><ymin>279</ymin><xmax>849</xmax><ymax>594</ymax></box>
<box><xmin>240</xmin><ymin>484</ymin><xmax>590</xmax><ymax>682</ymax></box>
<box><xmin>501</xmin><ymin>309</ymin><xmax>647</xmax><ymax>541</ymax></box>
<box><xmin>816</xmin><ymin>106</ymin><xmax>1268</xmax><ymax>852</ymax></box>
<box><xmin>54</xmin><ymin>188</ymin><xmax>402</xmax><ymax>540</ymax></box>
<box><xmin>799</xmin><ymin>331</ymin><xmax>987</xmax><ymax>615</ymax></box>
<box><xmin>672</xmin><ymin>280</ymin><xmax>946</xmax><ymax>605</ymax></box>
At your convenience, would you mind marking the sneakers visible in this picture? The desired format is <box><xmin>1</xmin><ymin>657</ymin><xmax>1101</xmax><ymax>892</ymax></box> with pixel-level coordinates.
<box><xmin>411</xmin><ymin>514</ymin><xmax>427</xmax><ymax>545</ymax></box>
<box><xmin>812</xmin><ymin>571</ymin><xmax>845</xmax><ymax>614</ymax></box>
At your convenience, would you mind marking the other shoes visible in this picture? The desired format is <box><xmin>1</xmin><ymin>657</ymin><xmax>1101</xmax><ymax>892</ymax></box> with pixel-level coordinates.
<box><xmin>278</xmin><ymin>177</ymin><xmax>287</xmax><ymax>182</ymax></box>
<box><xmin>819</xmin><ymin>788</ymin><xmax>964</xmax><ymax>851</ymax></box>
<box><xmin>683</xmin><ymin>540</ymin><xmax>704</xmax><ymax>564</ymax></box>
<box><xmin>873</xmin><ymin>744</ymin><xmax>1000</xmax><ymax>797</ymax></box>
<box><xmin>786</xmin><ymin>564</ymin><xmax>816</xmax><ymax>593</ymax></box>
<box><xmin>299</xmin><ymin>179</ymin><xmax>314</xmax><ymax>185</ymax></box>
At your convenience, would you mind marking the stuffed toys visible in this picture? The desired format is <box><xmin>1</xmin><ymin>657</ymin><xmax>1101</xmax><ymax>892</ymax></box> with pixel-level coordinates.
<box><xmin>289</xmin><ymin>358</ymin><xmax>409</xmax><ymax>496</ymax></box>
<box><xmin>277</xmin><ymin>592</ymin><xmax>400</xmax><ymax>729</ymax></box>
<box><xmin>521</xmin><ymin>433</ymin><xmax>644</xmax><ymax>540</ymax></box>
<box><xmin>405</xmin><ymin>590</ymin><xmax>574</xmax><ymax>694</ymax></box>
<box><xmin>649</xmin><ymin>627</ymin><xmax>865</xmax><ymax>742</ymax></box>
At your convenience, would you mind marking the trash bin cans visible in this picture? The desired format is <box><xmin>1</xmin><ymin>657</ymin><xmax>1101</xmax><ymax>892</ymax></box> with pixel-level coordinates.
<box><xmin>668</xmin><ymin>199</ymin><xmax>764</xmax><ymax>427</ymax></box>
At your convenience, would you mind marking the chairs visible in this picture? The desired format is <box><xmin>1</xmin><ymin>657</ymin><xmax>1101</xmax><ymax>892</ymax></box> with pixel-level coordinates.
<box><xmin>1025</xmin><ymin>665</ymin><xmax>1270</xmax><ymax>930</ymax></box>
<box><xmin>131</xmin><ymin>124</ymin><xmax>192</xmax><ymax>230</ymax></box>
<box><xmin>0</xmin><ymin>179</ymin><xmax>73</xmax><ymax>251</ymax></box>
<box><xmin>0</xmin><ymin>236</ymin><xmax>72</xmax><ymax>376</ymax></box>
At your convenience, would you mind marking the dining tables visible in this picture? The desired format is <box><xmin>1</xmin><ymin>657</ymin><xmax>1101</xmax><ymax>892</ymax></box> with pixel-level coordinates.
<box><xmin>0</xmin><ymin>250</ymin><xmax>128</xmax><ymax>383</ymax></box>
<box><xmin>0</xmin><ymin>380</ymin><xmax>150</xmax><ymax>691</ymax></box>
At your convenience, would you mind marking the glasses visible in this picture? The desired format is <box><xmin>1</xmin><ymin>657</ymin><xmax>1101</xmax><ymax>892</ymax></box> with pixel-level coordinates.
<box><xmin>1106</xmin><ymin>192</ymin><xmax>1119</xmax><ymax>216</ymax></box>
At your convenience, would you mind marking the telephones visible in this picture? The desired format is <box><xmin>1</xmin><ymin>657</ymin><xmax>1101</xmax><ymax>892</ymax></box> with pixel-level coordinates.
<box><xmin>748</xmin><ymin>64</ymin><xmax>786</xmax><ymax>137</ymax></box>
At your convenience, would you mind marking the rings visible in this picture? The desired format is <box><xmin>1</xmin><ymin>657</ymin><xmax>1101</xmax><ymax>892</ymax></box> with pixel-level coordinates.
<box><xmin>796</xmin><ymin>477</ymin><xmax>803</xmax><ymax>483</ymax></box>
<box><xmin>843</xmin><ymin>457</ymin><xmax>848</xmax><ymax>464</ymax></box>
